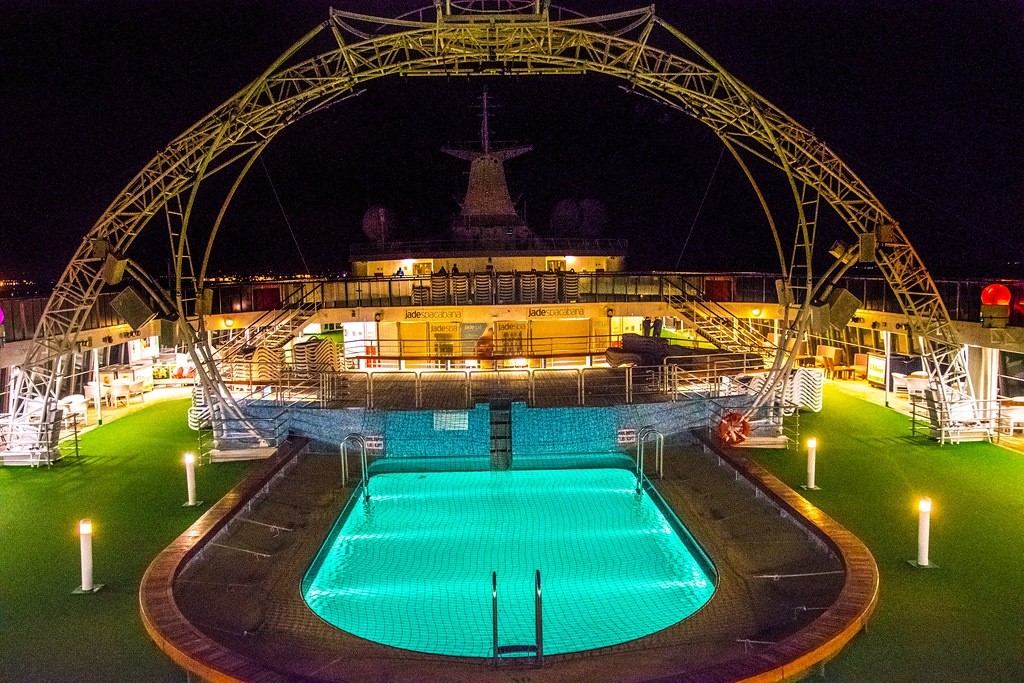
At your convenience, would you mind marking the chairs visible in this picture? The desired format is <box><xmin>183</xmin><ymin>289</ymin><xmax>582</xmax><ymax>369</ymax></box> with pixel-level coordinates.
<box><xmin>823</xmin><ymin>356</ymin><xmax>843</xmax><ymax>379</ymax></box>
<box><xmin>893</xmin><ymin>371</ymin><xmax>927</xmax><ymax>403</ymax></box>
<box><xmin>31</xmin><ymin>379</ymin><xmax>146</xmax><ymax>427</ymax></box>
<box><xmin>1000</xmin><ymin>397</ymin><xmax>1024</xmax><ymax>436</ymax></box>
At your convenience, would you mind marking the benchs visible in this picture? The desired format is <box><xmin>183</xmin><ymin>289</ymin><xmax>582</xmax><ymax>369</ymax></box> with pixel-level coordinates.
<box><xmin>853</xmin><ymin>353</ymin><xmax>868</xmax><ymax>378</ymax></box>
<box><xmin>767</xmin><ymin>333</ymin><xmax>842</xmax><ymax>366</ymax></box>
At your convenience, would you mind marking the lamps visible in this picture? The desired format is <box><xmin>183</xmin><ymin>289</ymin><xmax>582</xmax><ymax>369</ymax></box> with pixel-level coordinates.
<box><xmin>186</xmin><ymin>452</ymin><xmax>196</xmax><ymax>504</ymax></box>
<box><xmin>918</xmin><ymin>499</ymin><xmax>931</xmax><ymax>566</ymax></box>
<box><xmin>807</xmin><ymin>436</ymin><xmax>816</xmax><ymax>489</ymax></box>
<box><xmin>80</xmin><ymin>519</ymin><xmax>94</xmax><ymax>591</ymax></box>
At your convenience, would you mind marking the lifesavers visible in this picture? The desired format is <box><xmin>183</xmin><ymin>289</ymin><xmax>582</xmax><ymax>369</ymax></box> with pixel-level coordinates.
<box><xmin>719</xmin><ymin>414</ymin><xmax>749</xmax><ymax>445</ymax></box>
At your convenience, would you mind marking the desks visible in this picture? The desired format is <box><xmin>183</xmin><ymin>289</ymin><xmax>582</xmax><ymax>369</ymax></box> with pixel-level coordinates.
<box><xmin>103</xmin><ymin>385</ymin><xmax>125</xmax><ymax>406</ymax></box>
<box><xmin>902</xmin><ymin>375</ymin><xmax>928</xmax><ymax>401</ymax></box>
<box><xmin>843</xmin><ymin>365</ymin><xmax>856</xmax><ymax>379</ymax></box>
